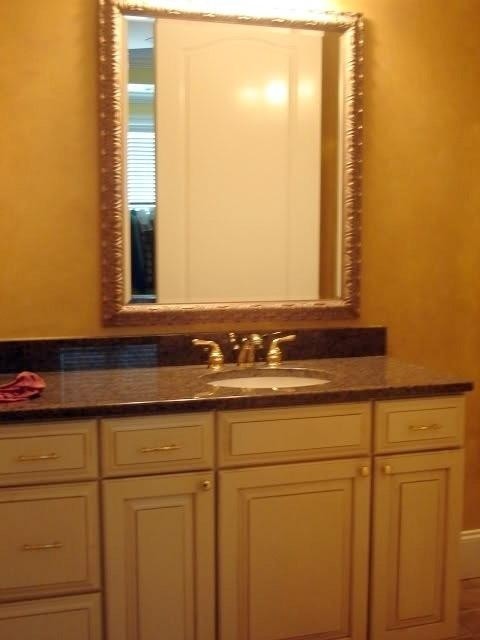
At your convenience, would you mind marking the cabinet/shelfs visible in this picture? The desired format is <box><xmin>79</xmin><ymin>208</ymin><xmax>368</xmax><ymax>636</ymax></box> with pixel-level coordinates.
<box><xmin>218</xmin><ymin>394</ymin><xmax>373</xmax><ymax>639</ymax></box>
<box><xmin>369</xmin><ymin>396</ymin><xmax>463</xmax><ymax>639</ymax></box>
<box><xmin>102</xmin><ymin>414</ymin><xmax>216</xmax><ymax>638</ymax></box>
<box><xmin>1</xmin><ymin>421</ymin><xmax>104</xmax><ymax>640</ymax></box>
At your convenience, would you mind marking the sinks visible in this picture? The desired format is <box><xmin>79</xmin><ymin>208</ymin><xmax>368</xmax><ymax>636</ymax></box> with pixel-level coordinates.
<box><xmin>199</xmin><ymin>368</ymin><xmax>335</xmax><ymax>389</ymax></box>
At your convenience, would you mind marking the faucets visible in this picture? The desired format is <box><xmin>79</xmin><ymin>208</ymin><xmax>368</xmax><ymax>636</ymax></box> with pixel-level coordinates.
<box><xmin>237</xmin><ymin>333</ymin><xmax>262</xmax><ymax>362</ymax></box>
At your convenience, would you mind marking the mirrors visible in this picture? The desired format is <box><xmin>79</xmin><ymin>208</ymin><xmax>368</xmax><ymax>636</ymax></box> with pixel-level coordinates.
<box><xmin>95</xmin><ymin>2</ymin><xmax>364</xmax><ymax>326</ymax></box>
